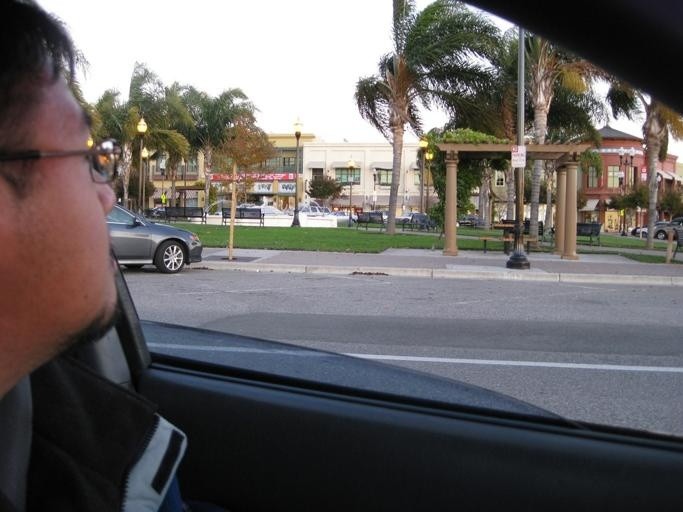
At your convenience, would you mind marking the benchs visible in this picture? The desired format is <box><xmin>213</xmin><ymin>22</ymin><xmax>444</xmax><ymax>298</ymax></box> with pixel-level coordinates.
<box><xmin>222</xmin><ymin>207</ymin><xmax>264</xmax><ymax>227</ymax></box>
<box><xmin>577</xmin><ymin>223</ymin><xmax>602</xmax><ymax>246</ymax></box>
<box><xmin>523</xmin><ymin>237</ymin><xmax>537</xmax><ymax>254</ymax></box>
<box><xmin>165</xmin><ymin>207</ymin><xmax>207</xmax><ymax>224</ymax></box>
<box><xmin>502</xmin><ymin>217</ymin><xmax>544</xmax><ymax>237</ymax></box>
<box><xmin>481</xmin><ymin>237</ymin><xmax>514</xmax><ymax>255</ymax></box>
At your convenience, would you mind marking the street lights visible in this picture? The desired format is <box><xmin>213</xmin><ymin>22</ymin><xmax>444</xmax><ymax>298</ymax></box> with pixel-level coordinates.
<box><xmin>418</xmin><ymin>136</ymin><xmax>433</xmax><ymax>215</ymax></box>
<box><xmin>348</xmin><ymin>160</ymin><xmax>356</xmax><ymax>227</ymax></box>
<box><xmin>137</xmin><ymin>116</ymin><xmax>149</xmax><ymax>213</ymax></box>
<box><xmin>292</xmin><ymin>119</ymin><xmax>304</xmax><ymax>226</ymax></box>
<box><xmin>618</xmin><ymin>146</ymin><xmax>636</xmax><ymax>235</ymax></box>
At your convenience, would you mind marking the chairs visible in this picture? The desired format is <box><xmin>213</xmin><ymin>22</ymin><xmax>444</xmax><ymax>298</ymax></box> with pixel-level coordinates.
<box><xmin>356</xmin><ymin>212</ymin><xmax>477</xmax><ymax>240</ymax></box>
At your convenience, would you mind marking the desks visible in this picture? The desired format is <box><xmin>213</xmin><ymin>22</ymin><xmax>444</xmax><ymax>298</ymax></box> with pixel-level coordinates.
<box><xmin>494</xmin><ymin>224</ymin><xmax>515</xmax><ymax>255</ymax></box>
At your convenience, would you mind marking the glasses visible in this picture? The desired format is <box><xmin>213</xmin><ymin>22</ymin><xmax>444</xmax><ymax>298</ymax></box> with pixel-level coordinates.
<box><xmin>0</xmin><ymin>138</ymin><xmax>119</xmax><ymax>183</ymax></box>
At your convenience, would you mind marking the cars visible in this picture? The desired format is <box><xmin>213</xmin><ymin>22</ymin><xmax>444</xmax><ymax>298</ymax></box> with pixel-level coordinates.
<box><xmin>638</xmin><ymin>218</ymin><xmax>683</xmax><ymax>245</ymax></box>
<box><xmin>290</xmin><ymin>205</ymin><xmax>358</xmax><ymax>223</ymax></box>
<box><xmin>106</xmin><ymin>203</ymin><xmax>202</xmax><ymax>272</ymax></box>
<box><xmin>376</xmin><ymin>210</ymin><xmax>389</xmax><ymax>221</ymax></box>
<box><xmin>239</xmin><ymin>202</ymin><xmax>283</xmax><ymax>216</ymax></box>
<box><xmin>399</xmin><ymin>211</ymin><xmax>428</xmax><ymax>222</ymax></box>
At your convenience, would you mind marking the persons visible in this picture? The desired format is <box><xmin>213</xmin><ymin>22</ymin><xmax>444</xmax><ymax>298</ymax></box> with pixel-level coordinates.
<box><xmin>0</xmin><ymin>0</ymin><xmax>188</xmax><ymax>509</ymax></box>
